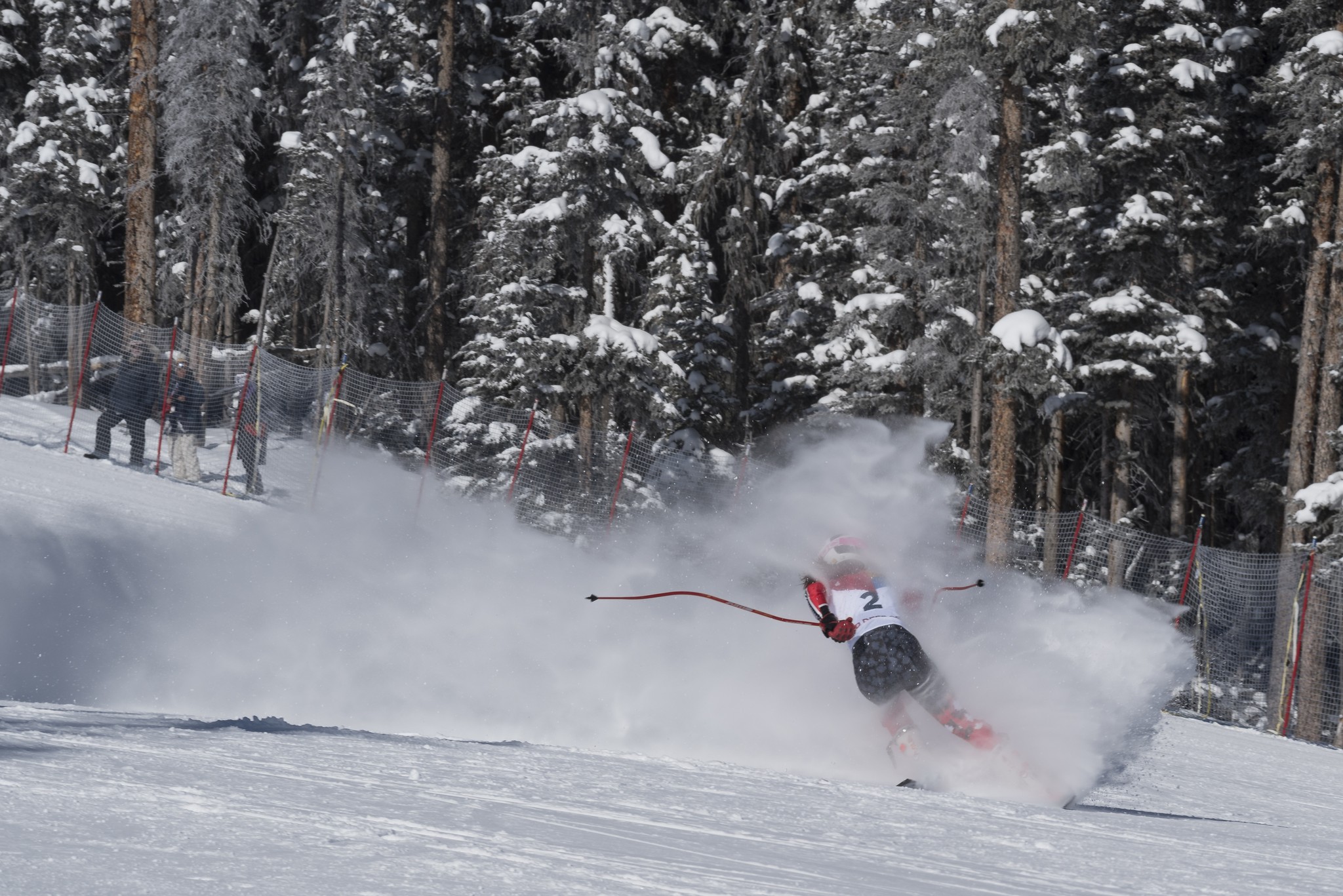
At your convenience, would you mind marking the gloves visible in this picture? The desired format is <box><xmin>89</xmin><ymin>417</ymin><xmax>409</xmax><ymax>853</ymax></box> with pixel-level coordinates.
<box><xmin>819</xmin><ymin>613</ymin><xmax>856</xmax><ymax>643</ymax></box>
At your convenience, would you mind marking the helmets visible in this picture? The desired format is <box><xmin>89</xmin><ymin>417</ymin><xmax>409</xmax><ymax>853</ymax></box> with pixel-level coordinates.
<box><xmin>819</xmin><ymin>533</ymin><xmax>870</xmax><ymax>570</ymax></box>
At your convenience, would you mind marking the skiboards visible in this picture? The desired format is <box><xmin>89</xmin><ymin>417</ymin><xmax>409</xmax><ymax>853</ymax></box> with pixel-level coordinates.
<box><xmin>896</xmin><ymin>778</ymin><xmax>1257</xmax><ymax>826</ymax></box>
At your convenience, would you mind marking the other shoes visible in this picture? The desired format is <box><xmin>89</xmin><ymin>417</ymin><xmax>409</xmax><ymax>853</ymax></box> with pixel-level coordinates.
<box><xmin>938</xmin><ymin>706</ymin><xmax>996</xmax><ymax>751</ymax></box>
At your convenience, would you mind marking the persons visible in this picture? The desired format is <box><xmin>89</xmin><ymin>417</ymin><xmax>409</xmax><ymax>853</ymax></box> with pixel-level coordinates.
<box><xmin>236</xmin><ymin>371</ymin><xmax>270</xmax><ymax>493</ymax></box>
<box><xmin>803</xmin><ymin>532</ymin><xmax>999</xmax><ymax>769</ymax></box>
<box><xmin>166</xmin><ymin>359</ymin><xmax>206</xmax><ymax>480</ymax></box>
<box><xmin>82</xmin><ymin>335</ymin><xmax>160</xmax><ymax>465</ymax></box>
